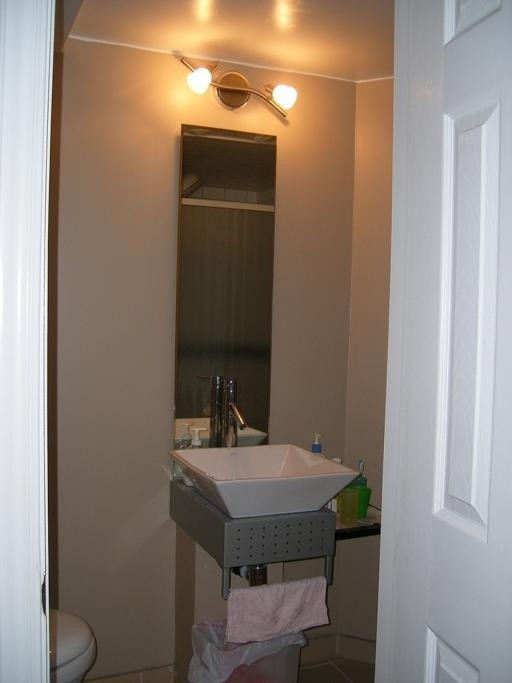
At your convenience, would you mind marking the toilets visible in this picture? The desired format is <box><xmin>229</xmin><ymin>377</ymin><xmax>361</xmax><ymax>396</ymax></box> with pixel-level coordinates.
<box><xmin>50</xmin><ymin>607</ymin><xmax>98</xmax><ymax>682</ymax></box>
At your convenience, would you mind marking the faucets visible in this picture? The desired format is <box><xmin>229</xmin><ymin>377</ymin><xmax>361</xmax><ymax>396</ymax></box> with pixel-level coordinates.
<box><xmin>194</xmin><ymin>376</ymin><xmax>212</xmax><ymax>384</ymax></box>
<box><xmin>210</xmin><ymin>375</ymin><xmax>247</xmax><ymax>447</ymax></box>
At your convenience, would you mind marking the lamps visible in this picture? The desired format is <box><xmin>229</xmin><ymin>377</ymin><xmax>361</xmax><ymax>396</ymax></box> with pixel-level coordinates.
<box><xmin>180</xmin><ymin>55</ymin><xmax>298</xmax><ymax>117</ymax></box>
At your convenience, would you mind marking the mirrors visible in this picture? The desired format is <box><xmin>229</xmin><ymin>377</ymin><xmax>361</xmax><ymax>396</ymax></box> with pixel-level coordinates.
<box><xmin>173</xmin><ymin>123</ymin><xmax>277</xmax><ymax>449</ymax></box>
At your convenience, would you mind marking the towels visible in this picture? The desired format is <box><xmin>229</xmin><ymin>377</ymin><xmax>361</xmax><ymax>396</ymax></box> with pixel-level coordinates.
<box><xmin>224</xmin><ymin>576</ymin><xmax>330</xmax><ymax>645</ymax></box>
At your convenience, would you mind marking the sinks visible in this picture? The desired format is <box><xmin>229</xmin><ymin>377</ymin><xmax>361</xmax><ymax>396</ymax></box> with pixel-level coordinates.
<box><xmin>175</xmin><ymin>417</ymin><xmax>268</xmax><ymax>448</ymax></box>
<box><xmin>171</xmin><ymin>443</ymin><xmax>361</xmax><ymax>519</ymax></box>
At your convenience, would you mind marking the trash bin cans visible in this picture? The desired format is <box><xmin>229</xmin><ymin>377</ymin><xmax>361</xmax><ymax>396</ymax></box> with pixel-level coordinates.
<box><xmin>192</xmin><ymin>617</ymin><xmax>301</xmax><ymax>683</ymax></box>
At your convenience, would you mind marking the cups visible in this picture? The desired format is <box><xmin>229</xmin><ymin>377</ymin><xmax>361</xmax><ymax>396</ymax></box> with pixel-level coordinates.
<box><xmin>337</xmin><ymin>485</ymin><xmax>371</xmax><ymax>524</ymax></box>
